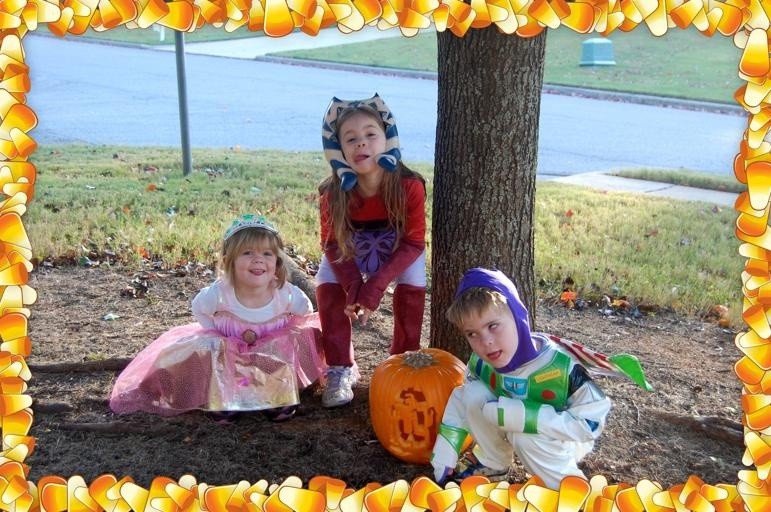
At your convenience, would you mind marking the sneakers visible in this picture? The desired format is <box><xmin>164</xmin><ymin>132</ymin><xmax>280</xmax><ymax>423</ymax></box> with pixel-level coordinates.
<box><xmin>211</xmin><ymin>411</ymin><xmax>239</xmax><ymax>426</ymax></box>
<box><xmin>453</xmin><ymin>458</ymin><xmax>511</xmax><ymax>482</ymax></box>
<box><xmin>319</xmin><ymin>358</ymin><xmax>362</xmax><ymax>408</ymax></box>
<box><xmin>268</xmin><ymin>406</ymin><xmax>297</xmax><ymax>421</ymax></box>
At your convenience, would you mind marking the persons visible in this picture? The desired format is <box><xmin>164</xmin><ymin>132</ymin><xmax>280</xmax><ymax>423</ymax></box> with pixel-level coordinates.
<box><xmin>428</xmin><ymin>267</ymin><xmax>653</xmax><ymax>494</ymax></box>
<box><xmin>308</xmin><ymin>91</ymin><xmax>428</xmax><ymax>409</ymax></box>
<box><xmin>110</xmin><ymin>214</ymin><xmax>329</xmax><ymax>423</ymax></box>
